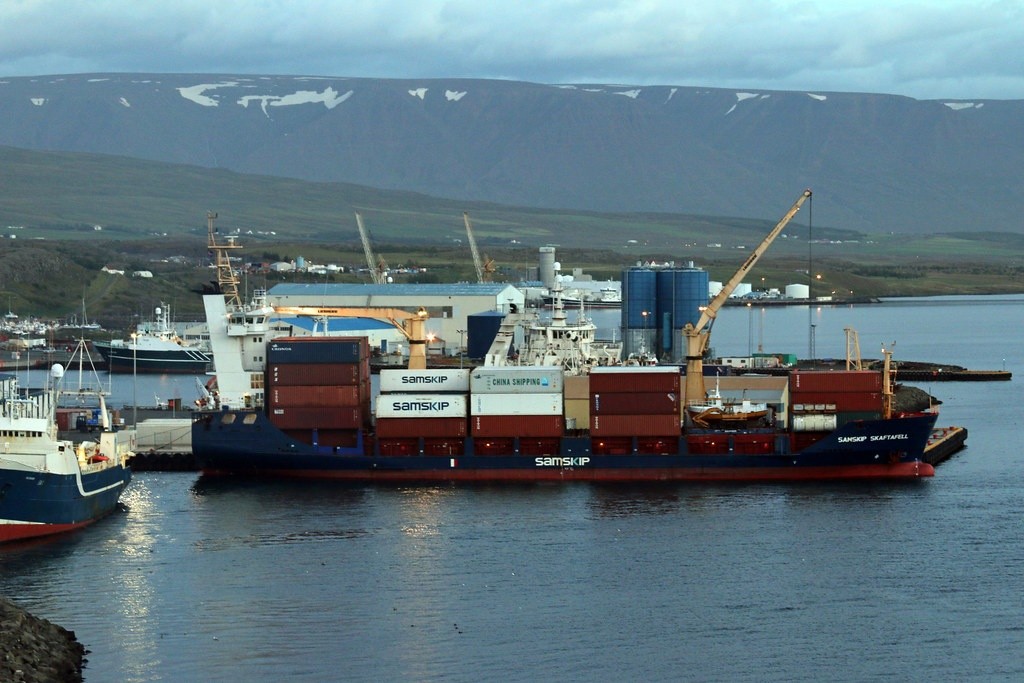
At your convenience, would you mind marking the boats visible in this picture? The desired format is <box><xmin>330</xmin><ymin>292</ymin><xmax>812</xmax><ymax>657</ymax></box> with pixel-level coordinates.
<box><xmin>686</xmin><ymin>367</ymin><xmax>769</xmax><ymax>428</ymax></box>
<box><xmin>190</xmin><ymin>261</ymin><xmax>935</xmax><ymax>479</ymax></box>
<box><xmin>91</xmin><ymin>296</ymin><xmax>211</xmax><ymax>373</ymax></box>
<box><xmin>0</xmin><ymin>296</ymin><xmax>106</xmax><ymax>354</ymax></box>
<box><xmin>0</xmin><ymin>328</ymin><xmax>131</xmax><ymax>542</ymax></box>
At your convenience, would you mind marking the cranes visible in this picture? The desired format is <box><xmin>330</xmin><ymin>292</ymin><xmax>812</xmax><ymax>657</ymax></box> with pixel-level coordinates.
<box><xmin>356</xmin><ymin>210</ymin><xmax>388</xmax><ymax>285</ymax></box>
<box><xmin>462</xmin><ymin>211</ymin><xmax>496</xmax><ymax>283</ymax></box>
<box><xmin>684</xmin><ymin>188</ymin><xmax>815</xmax><ymax>402</ymax></box>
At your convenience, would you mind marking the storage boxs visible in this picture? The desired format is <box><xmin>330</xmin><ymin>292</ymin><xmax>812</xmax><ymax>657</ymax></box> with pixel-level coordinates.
<box><xmin>56</xmin><ymin>408</ymin><xmax>121</xmax><ymax>431</ymax></box>
<box><xmin>262</xmin><ymin>336</ymin><xmax>887</xmax><ymax>456</ymax></box>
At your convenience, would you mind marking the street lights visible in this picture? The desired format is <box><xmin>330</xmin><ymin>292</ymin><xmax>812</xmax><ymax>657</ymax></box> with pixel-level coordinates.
<box><xmin>455</xmin><ymin>329</ymin><xmax>467</xmax><ymax>369</ymax></box>
<box><xmin>131</xmin><ymin>333</ymin><xmax>136</xmax><ymax>430</ymax></box>
